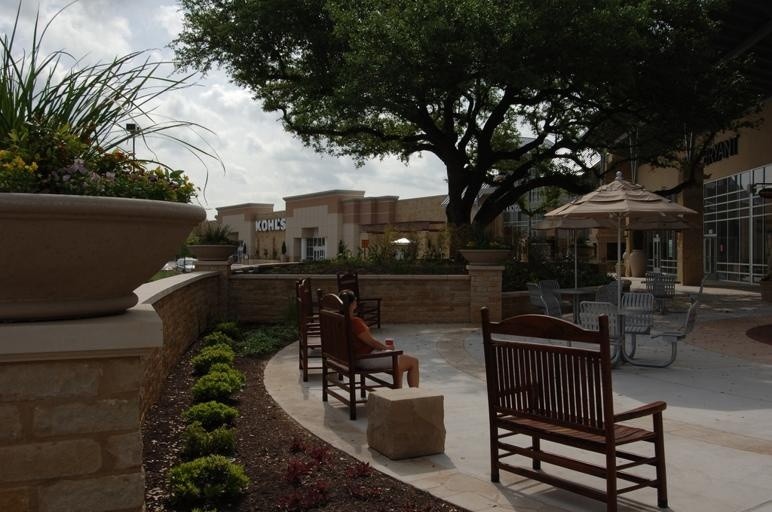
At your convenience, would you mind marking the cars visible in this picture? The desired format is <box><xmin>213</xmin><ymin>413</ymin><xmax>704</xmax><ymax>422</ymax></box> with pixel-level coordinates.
<box><xmin>385</xmin><ymin>338</ymin><xmax>393</xmax><ymax>346</ymax></box>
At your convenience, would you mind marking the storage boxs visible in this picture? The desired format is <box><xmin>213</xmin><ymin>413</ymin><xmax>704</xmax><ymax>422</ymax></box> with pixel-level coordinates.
<box><xmin>479</xmin><ymin>307</ymin><xmax>668</xmax><ymax>511</ymax></box>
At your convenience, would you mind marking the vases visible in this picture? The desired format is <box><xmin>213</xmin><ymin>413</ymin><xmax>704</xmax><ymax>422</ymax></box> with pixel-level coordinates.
<box><xmin>1</xmin><ymin>0</ymin><xmax>229</xmax><ymax>204</ymax></box>
<box><xmin>461</xmin><ymin>219</ymin><xmax>511</xmax><ymax>250</ymax></box>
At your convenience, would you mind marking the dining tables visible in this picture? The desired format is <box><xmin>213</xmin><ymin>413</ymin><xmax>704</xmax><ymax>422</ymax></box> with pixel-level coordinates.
<box><xmin>187</xmin><ymin>220</ymin><xmax>238</xmax><ymax>261</ymax></box>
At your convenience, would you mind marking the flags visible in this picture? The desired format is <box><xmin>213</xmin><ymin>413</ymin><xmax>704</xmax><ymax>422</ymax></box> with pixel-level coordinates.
<box><xmin>460</xmin><ymin>248</ymin><xmax>502</xmax><ymax>266</ymax></box>
<box><xmin>0</xmin><ymin>190</ymin><xmax>211</xmax><ymax>326</ymax></box>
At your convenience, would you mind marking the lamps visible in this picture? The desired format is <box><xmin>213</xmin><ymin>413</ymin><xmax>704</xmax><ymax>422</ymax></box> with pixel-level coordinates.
<box><xmin>537</xmin><ymin>271</ymin><xmax>708</xmax><ymax>372</ymax></box>
<box><xmin>293</xmin><ymin>269</ymin><xmax>403</xmax><ymax>419</ymax></box>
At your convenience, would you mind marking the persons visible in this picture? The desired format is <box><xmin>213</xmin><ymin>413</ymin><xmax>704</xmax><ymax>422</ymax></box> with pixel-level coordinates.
<box><xmin>338</xmin><ymin>289</ymin><xmax>419</xmax><ymax>387</ymax></box>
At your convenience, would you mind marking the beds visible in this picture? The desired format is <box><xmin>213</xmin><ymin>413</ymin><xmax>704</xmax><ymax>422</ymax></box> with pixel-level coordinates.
<box><xmin>544</xmin><ymin>171</ymin><xmax>698</xmax><ymax>316</ymax></box>
<box><xmin>528</xmin><ymin>216</ymin><xmax>618</xmax><ymax>324</ymax></box>
<box><xmin>622</xmin><ymin>214</ymin><xmax>695</xmax><ymax>276</ymax></box>
<box><xmin>389</xmin><ymin>238</ymin><xmax>416</xmax><ymax>246</ymax></box>
